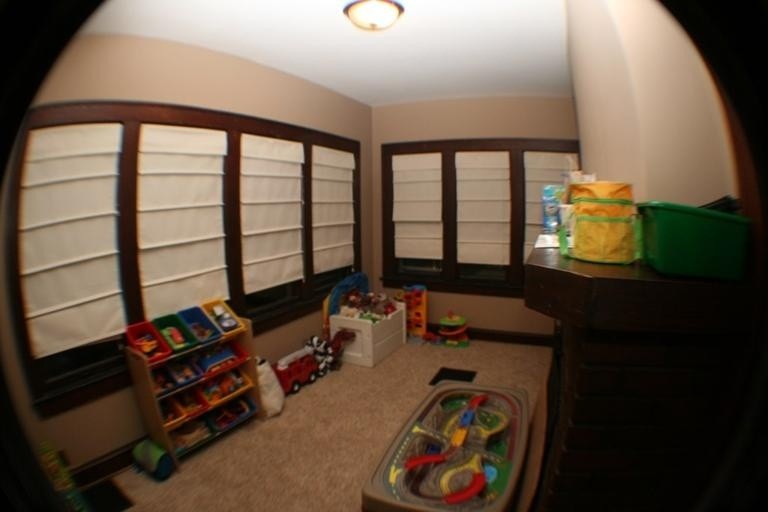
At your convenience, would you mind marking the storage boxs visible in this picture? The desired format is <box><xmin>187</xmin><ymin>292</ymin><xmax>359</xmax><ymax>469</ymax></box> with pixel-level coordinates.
<box><xmin>330</xmin><ymin>300</ymin><xmax>408</xmax><ymax>369</ymax></box>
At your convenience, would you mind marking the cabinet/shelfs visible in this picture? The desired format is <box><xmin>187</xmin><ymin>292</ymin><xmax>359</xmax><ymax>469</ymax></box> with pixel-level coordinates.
<box><xmin>119</xmin><ymin>316</ymin><xmax>267</xmax><ymax>459</ymax></box>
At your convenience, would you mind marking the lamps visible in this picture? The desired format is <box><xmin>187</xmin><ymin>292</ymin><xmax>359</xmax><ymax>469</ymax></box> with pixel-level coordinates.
<box><xmin>342</xmin><ymin>1</ymin><xmax>406</xmax><ymax>32</ymax></box>
